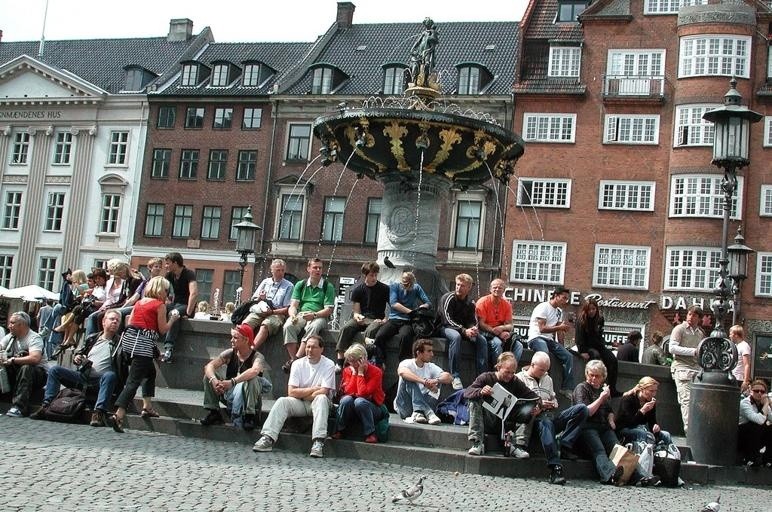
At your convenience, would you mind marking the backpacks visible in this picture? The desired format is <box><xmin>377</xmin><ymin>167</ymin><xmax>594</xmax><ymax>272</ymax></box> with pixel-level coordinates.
<box><xmin>231</xmin><ymin>298</ymin><xmax>277</xmax><ymax>324</ymax></box>
<box><xmin>408</xmin><ymin>307</ymin><xmax>442</xmax><ymax>339</ymax></box>
<box><xmin>44</xmin><ymin>387</ymin><xmax>86</xmax><ymax>422</ymax></box>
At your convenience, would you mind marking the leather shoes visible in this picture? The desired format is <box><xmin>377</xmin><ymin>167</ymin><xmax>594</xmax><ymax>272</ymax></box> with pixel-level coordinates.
<box><xmin>365</xmin><ymin>434</ymin><xmax>377</xmax><ymax>443</ymax></box>
<box><xmin>332</xmin><ymin>431</ymin><xmax>342</xmax><ymax>439</ymax></box>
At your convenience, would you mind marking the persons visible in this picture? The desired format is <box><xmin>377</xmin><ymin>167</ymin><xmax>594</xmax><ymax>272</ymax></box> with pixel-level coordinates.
<box><xmin>410</xmin><ymin>18</ymin><xmax>437</xmax><ymax>74</ymax></box>
<box><xmin>0</xmin><ymin>251</ymin><xmax>198</xmax><ymax>435</ymax></box>
<box><xmin>201</xmin><ymin>254</ymin><xmax>566</xmax><ymax>482</ymax></box>
<box><xmin>671</xmin><ymin>306</ymin><xmax>772</xmax><ymax>487</ymax></box>
<box><xmin>574</xmin><ymin>360</ymin><xmax>687</xmax><ymax>488</ymax></box>
<box><xmin>528</xmin><ymin>286</ymin><xmax>669</xmax><ymax>393</ymax></box>
<box><xmin>410</xmin><ymin>52</ymin><xmax>420</xmax><ymax>75</ymax></box>
<box><xmin>426</xmin><ymin>25</ymin><xmax>440</xmax><ymax>44</ymax></box>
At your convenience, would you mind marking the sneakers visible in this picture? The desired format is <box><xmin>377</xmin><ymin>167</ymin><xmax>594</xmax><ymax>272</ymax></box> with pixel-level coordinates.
<box><xmin>467</xmin><ymin>442</ymin><xmax>578</xmax><ymax>485</ymax></box>
<box><xmin>6</xmin><ymin>406</ymin><xmax>23</xmax><ymax>417</ymax></box>
<box><xmin>310</xmin><ymin>440</ymin><xmax>324</xmax><ymax>457</ymax></box>
<box><xmin>286</xmin><ymin>356</ymin><xmax>299</xmax><ymax>368</ymax></box>
<box><xmin>636</xmin><ymin>475</ymin><xmax>661</xmax><ymax>487</ymax></box>
<box><xmin>282</xmin><ymin>365</ymin><xmax>289</xmax><ymax>373</ymax></box>
<box><xmin>253</xmin><ymin>436</ymin><xmax>272</xmax><ymax>452</ymax></box>
<box><xmin>610</xmin><ymin>465</ymin><xmax>624</xmax><ymax>486</ymax></box>
<box><xmin>678</xmin><ymin>477</ymin><xmax>685</xmax><ymax>486</ymax></box>
<box><xmin>200</xmin><ymin>411</ymin><xmax>224</xmax><ymax>425</ymax></box>
<box><xmin>334</xmin><ymin>364</ymin><xmax>342</xmax><ymax>373</ymax></box>
<box><xmin>412</xmin><ymin>378</ymin><xmax>463</xmax><ymax>424</ymax></box>
<box><xmin>244</xmin><ymin>415</ymin><xmax>255</xmax><ymax>430</ymax></box>
<box><xmin>29</xmin><ymin>406</ymin><xmax>45</xmax><ymax>419</ymax></box>
<box><xmin>90</xmin><ymin>411</ymin><xmax>103</xmax><ymax>426</ymax></box>
<box><xmin>161</xmin><ymin>350</ymin><xmax>175</xmax><ymax>363</ymax></box>
<box><xmin>558</xmin><ymin>390</ymin><xmax>573</xmax><ymax>399</ymax></box>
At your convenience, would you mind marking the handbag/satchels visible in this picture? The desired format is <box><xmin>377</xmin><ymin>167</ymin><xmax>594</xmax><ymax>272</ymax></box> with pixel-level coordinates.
<box><xmin>152</xmin><ymin>345</ymin><xmax>160</xmax><ymax>358</ymax></box>
<box><xmin>609</xmin><ymin>443</ymin><xmax>640</xmax><ymax>486</ymax></box>
<box><xmin>654</xmin><ymin>443</ymin><xmax>681</xmax><ymax>487</ymax></box>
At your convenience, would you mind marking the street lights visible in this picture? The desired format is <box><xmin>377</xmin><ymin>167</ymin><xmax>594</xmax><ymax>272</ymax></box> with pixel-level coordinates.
<box><xmin>681</xmin><ymin>73</ymin><xmax>764</xmax><ymax>486</ymax></box>
<box><xmin>231</xmin><ymin>202</ymin><xmax>264</xmax><ymax>287</ymax></box>
<box><xmin>726</xmin><ymin>220</ymin><xmax>755</xmax><ymax>332</ymax></box>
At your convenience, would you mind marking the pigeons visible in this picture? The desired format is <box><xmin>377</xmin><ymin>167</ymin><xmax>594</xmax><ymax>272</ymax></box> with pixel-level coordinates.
<box><xmin>700</xmin><ymin>495</ymin><xmax>722</xmax><ymax>512</ymax></box>
<box><xmin>391</xmin><ymin>474</ymin><xmax>428</xmax><ymax>506</ymax></box>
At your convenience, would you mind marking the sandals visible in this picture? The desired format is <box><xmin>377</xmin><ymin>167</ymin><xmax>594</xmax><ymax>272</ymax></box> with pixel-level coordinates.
<box><xmin>141</xmin><ymin>408</ymin><xmax>159</xmax><ymax>418</ymax></box>
<box><xmin>109</xmin><ymin>414</ymin><xmax>125</xmax><ymax>433</ymax></box>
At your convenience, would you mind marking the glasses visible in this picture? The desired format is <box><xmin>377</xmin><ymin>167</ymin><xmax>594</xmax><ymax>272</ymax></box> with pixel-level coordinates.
<box><xmin>753</xmin><ymin>389</ymin><xmax>765</xmax><ymax>393</ymax></box>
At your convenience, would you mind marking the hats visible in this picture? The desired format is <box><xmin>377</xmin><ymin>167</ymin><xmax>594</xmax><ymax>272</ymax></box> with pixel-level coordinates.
<box><xmin>236</xmin><ymin>323</ymin><xmax>254</xmax><ymax>346</ymax></box>
